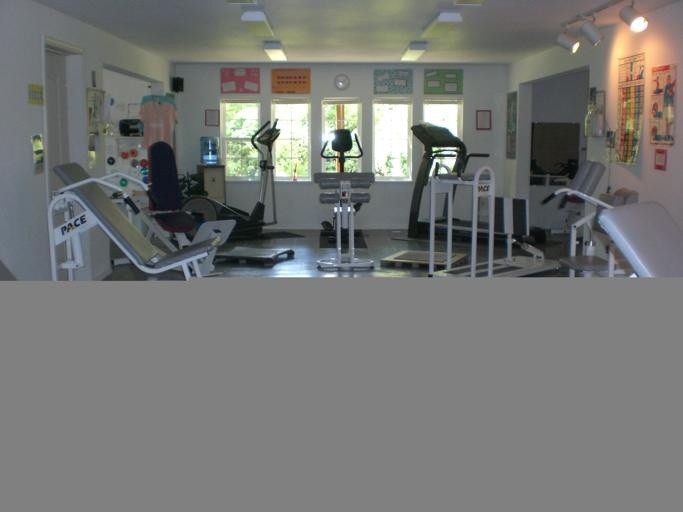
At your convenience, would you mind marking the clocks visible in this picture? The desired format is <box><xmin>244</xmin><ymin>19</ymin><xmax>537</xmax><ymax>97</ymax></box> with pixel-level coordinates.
<box><xmin>335</xmin><ymin>73</ymin><xmax>350</xmax><ymax>90</ymax></box>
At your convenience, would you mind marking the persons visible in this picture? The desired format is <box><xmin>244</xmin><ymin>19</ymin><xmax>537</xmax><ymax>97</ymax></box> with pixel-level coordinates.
<box><xmin>653</xmin><ymin>76</ymin><xmax>659</xmax><ymax>93</ymax></box>
<box><xmin>650</xmin><ymin>102</ymin><xmax>658</xmax><ymax>140</ymax></box>
<box><xmin>663</xmin><ymin>74</ymin><xmax>676</xmax><ymax>136</ymax></box>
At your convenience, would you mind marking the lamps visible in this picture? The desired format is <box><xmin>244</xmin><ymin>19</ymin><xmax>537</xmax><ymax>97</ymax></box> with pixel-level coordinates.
<box><xmin>556</xmin><ymin>0</ymin><xmax>648</xmax><ymax>53</ymax></box>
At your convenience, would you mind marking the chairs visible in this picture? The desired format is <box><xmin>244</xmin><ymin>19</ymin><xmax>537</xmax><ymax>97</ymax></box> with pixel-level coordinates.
<box><xmin>47</xmin><ymin>162</ymin><xmax>222</xmax><ymax>281</ymax></box>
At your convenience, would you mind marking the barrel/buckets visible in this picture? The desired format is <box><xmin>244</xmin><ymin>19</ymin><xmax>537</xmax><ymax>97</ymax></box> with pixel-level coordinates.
<box><xmin>200</xmin><ymin>136</ymin><xmax>219</xmax><ymax>165</ymax></box>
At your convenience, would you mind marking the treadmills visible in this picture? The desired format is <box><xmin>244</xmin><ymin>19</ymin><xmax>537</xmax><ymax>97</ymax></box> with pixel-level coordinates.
<box><xmin>407</xmin><ymin>123</ymin><xmax>547</xmax><ymax>250</ymax></box>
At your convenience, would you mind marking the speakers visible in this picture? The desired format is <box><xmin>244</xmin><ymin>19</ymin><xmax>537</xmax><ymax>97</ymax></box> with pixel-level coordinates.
<box><xmin>172</xmin><ymin>77</ymin><xmax>184</xmax><ymax>92</ymax></box>
